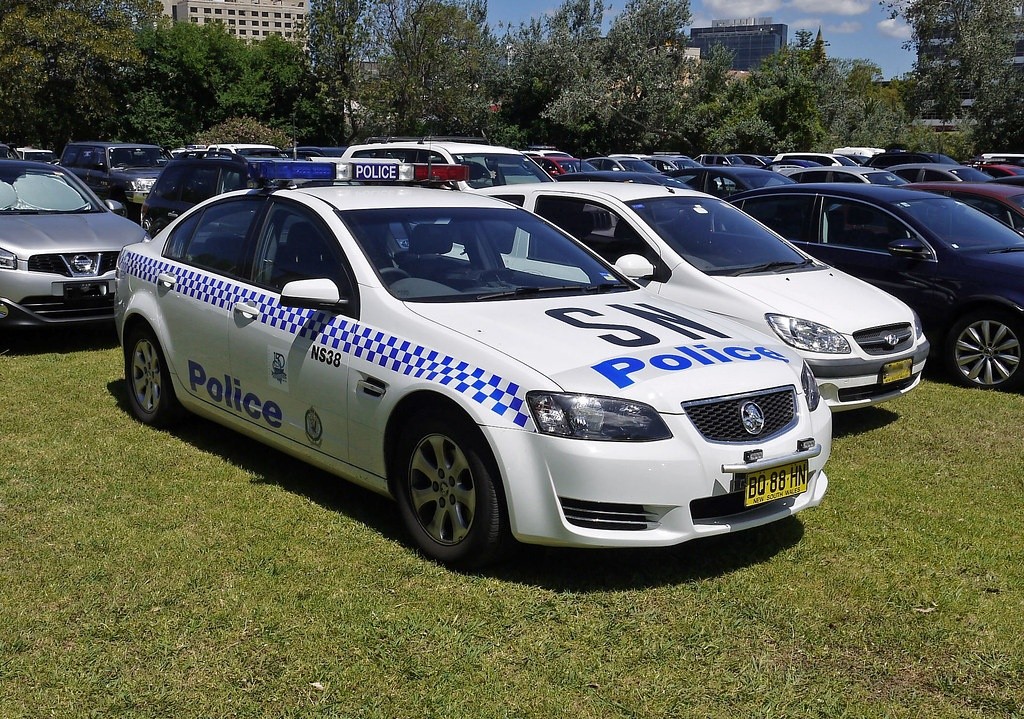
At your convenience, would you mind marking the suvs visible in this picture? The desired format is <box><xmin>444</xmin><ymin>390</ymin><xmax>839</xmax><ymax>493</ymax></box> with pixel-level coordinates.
<box><xmin>59</xmin><ymin>142</ymin><xmax>175</xmax><ymax>219</ymax></box>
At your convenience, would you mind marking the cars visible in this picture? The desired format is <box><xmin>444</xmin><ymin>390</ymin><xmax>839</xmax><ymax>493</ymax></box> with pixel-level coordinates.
<box><xmin>0</xmin><ymin>143</ymin><xmax>153</xmax><ymax>352</ymax></box>
<box><xmin>663</xmin><ymin>180</ymin><xmax>1024</xmax><ymax>392</ymax></box>
<box><xmin>113</xmin><ymin>157</ymin><xmax>832</xmax><ymax>577</ymax></box>
<box><xmin>142</xmin><ymin>136</ymin><xmax>1024</xmax><ymax>240</ymax></box>
<box><xmin>413</xmin><ymin>180</ymin><xmax>931</xmax><ymax>413</ymax></box>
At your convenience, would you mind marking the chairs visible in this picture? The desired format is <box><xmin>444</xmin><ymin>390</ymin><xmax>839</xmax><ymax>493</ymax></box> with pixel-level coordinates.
<box><xmin>558</xmin><ymin>210</ymin><xmax>610</xmax><ymax>262</ymax></box>
<box><xmin>274</xmin><ymin>223</ymin><xmax>340</xmax><ymax>295</ymax></box>
<box><xmin>407</xmin><ymin>224</ymin><xmax>476</xmax><ymax>290</ymax></box>
<box><xmin>613</xmin><ymin>214</ymin><xmax>667</xmax><ymax>271</ymax></box>
